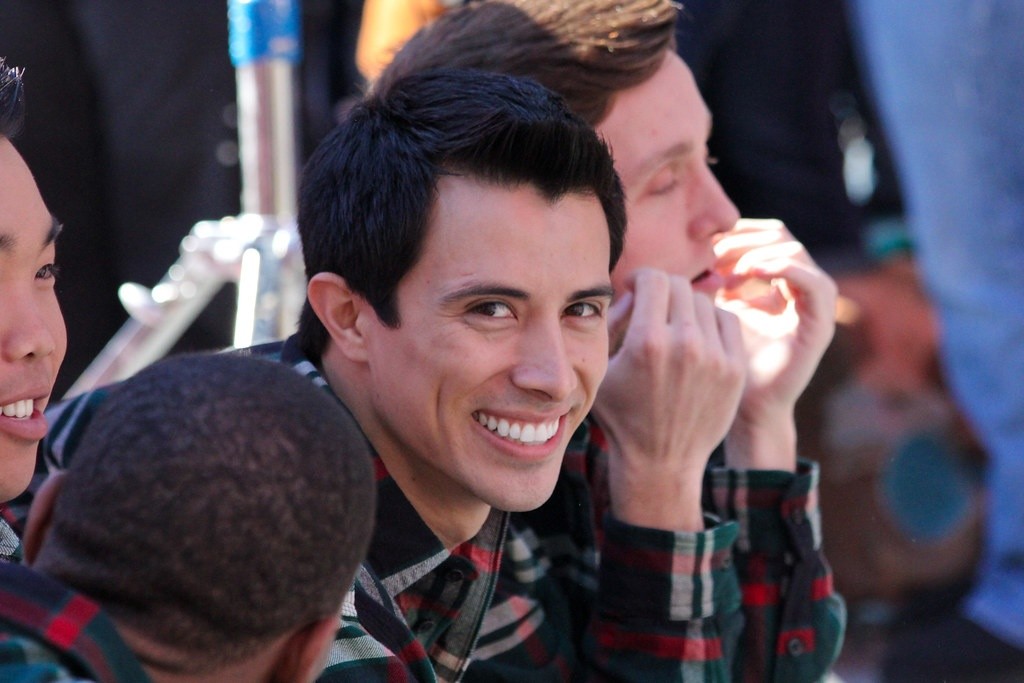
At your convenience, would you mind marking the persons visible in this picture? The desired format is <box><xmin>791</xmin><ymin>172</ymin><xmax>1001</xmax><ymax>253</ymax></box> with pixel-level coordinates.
<box><xmin>1</xmin><ymin>61</ymin><xmax>68</xmax><ymax>562</ymax></box>
<box><xmin>810</xmin><ymin>298</ymin><xmax>982</xmax><ymax>622</ymax></box>
<box><xmin>369</xmin><ymin>1</ymin><xmax>847</xmax><ymax>683</ymax></box>
<box><xmin>4</xmin><ymin>70</ymin><xmax>628</xmax><ymax>681</ymax></box>
<box><xmin>854</xmin><ymin>0</ymin><xmax>1024</xmax><ymax>683</ymax></box>
<box><xmin>0</xmin><ymin>350</ymin><xmax>376</xmax><ymax>682</ymax></box>
